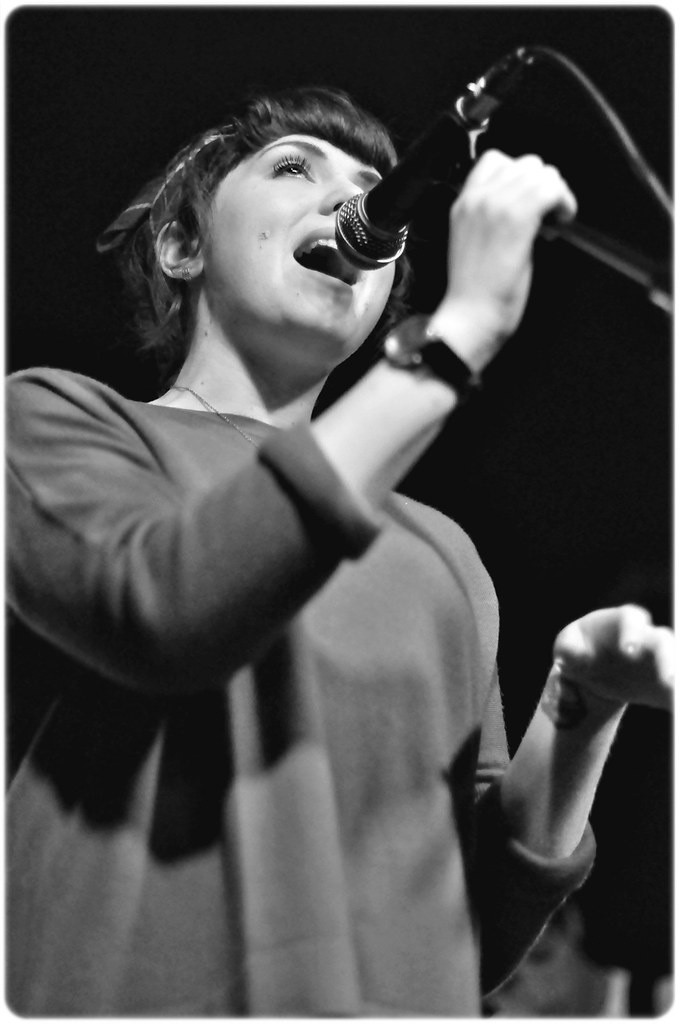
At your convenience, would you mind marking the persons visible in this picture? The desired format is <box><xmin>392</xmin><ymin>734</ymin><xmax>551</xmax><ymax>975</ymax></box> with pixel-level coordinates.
<box><xmin>4</xmin><ymin>72</ymin><xmax>676</xmax><ymax>1019</ymax></box>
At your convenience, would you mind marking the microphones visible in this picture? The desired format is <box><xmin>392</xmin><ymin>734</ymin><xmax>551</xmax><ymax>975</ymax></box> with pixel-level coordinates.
<box><xmin>335</xmin><ymin>48</ymin><xmax>536</xmax><ymax>269</ymax></box>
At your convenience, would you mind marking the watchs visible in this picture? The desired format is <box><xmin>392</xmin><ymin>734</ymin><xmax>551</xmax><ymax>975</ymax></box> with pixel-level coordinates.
<box><xmin>380</xmin><ymin>308</ymin><xmax>484</xmax><ymax>405</ymax></box>
